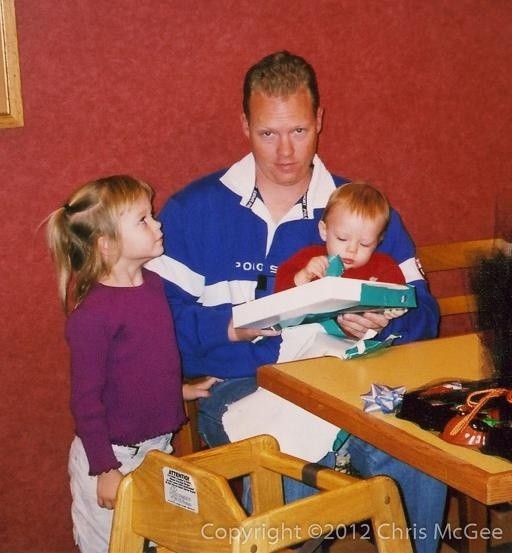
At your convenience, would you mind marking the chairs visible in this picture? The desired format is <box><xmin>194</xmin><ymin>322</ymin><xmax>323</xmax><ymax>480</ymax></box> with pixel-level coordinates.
<box><xmin>105</xmin><ymin>432</ymin><xmax>417</xmax><ymax>553</ymax></box>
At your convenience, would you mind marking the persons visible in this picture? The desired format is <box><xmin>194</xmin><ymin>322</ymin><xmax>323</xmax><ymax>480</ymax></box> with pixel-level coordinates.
<box><xmin>35</xmin><ymin>175</ymin><xmax>223</xmax><ymax>552</ymax></box>
<box><xmin>156</xmin><ymin>48</ymin><xmax>447</xmax><ymax>551</ymax></box>
<box><xmin>273</xmin><ymin>181</ymin><xmax>408</xmax><ymax>320</ymax></box>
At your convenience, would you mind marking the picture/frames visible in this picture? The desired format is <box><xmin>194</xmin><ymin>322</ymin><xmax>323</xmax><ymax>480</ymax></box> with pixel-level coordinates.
<box><xmin>1</xmin><ymin>1</ymin><xmax>26</xmax><ymax>129</ymax></box>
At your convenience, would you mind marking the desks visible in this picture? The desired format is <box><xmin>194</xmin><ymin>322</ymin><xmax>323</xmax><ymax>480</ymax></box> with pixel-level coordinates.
<box><xmin>255</xmin><ymin>332</ymin><xmax>511</xmax><ymax>551</ymax></box>
